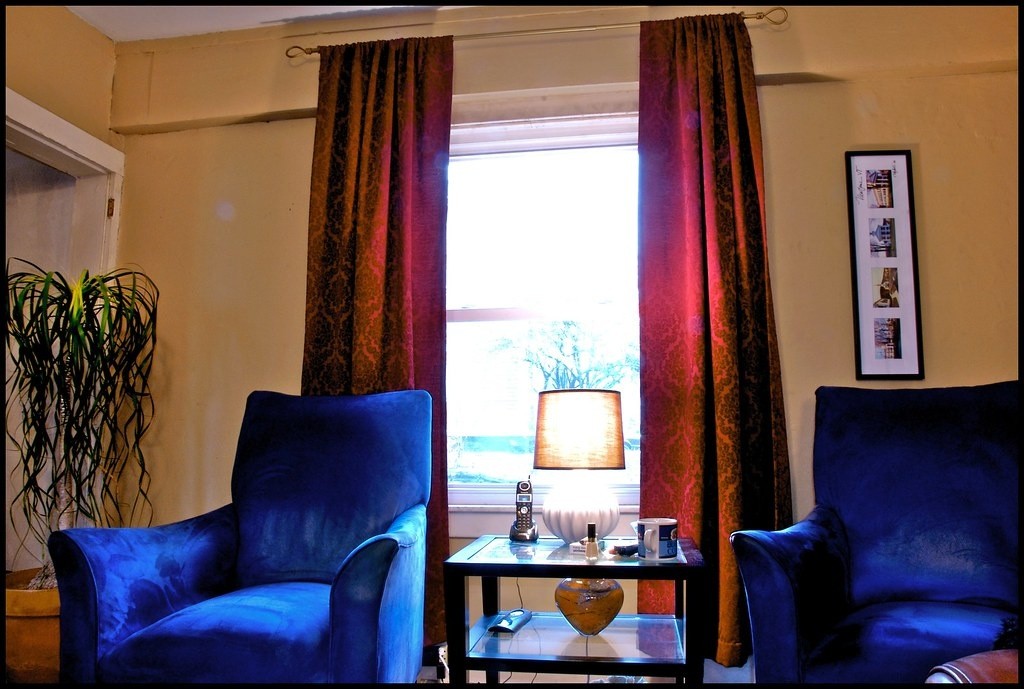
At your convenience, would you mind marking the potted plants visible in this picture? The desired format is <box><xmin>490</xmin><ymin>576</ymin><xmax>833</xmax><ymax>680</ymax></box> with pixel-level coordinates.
<box><xmin>6</xmin><ymin>258</ymin><xmax>161</xmax><ymax>682</ymax></box>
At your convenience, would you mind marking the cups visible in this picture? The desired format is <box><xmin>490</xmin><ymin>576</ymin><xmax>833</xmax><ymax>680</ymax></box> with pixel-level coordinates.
<box><xmin>637</xmin><ymin>518</ymin><xmax>678</xmax><ymax>560</ymax></box>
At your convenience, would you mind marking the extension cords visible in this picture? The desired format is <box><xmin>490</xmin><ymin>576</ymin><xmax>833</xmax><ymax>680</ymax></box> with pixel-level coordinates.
<box><xmin>437</xmin><ymin>641</ymin><xmax>449</xmax><ymax>684</ymax></box>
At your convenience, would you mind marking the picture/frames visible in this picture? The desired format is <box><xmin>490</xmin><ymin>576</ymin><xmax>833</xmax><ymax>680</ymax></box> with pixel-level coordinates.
<box><xmin>844</xmin><ymin>150</ymin><xmax>925</xmax><ymax>380</ymax></box>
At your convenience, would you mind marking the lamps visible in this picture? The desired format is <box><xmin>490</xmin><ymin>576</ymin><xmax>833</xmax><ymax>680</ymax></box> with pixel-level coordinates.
<box><xmin>534</xmin><ymin>388</ymin><xmax>627</xmax><ymax>543</ymax></box>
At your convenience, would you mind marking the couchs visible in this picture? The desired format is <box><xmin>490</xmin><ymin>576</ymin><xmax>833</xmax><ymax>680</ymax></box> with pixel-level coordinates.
<box><xmin>45</xmin><ymin>390</ymin><xmax>437</xmax><ymax>688</ymax></box>
<box><xmin>730</xmin><ymin>380</ymin><xmax>1023</xmax><ymax>685</ymax></box>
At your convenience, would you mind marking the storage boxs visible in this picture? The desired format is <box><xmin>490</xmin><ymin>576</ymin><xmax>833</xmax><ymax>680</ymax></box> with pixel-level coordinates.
<box><xmin>567</xmin><ymin>538</ymin><xmax>604</xmax><ymax>555</ymax></box>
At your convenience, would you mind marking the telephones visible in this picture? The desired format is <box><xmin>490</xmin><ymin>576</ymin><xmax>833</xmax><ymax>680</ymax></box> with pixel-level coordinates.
<box><xmin>509</xmin><ymin>474</ymin><xmax>539</xmax><ymax>541</ymax></box>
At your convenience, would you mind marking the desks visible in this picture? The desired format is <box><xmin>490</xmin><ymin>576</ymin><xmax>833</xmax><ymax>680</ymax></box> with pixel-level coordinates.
<box><xmin>445</xmin><ymin>534</ymin><xmax>707</xmax><ymax>683</ymax></box>
